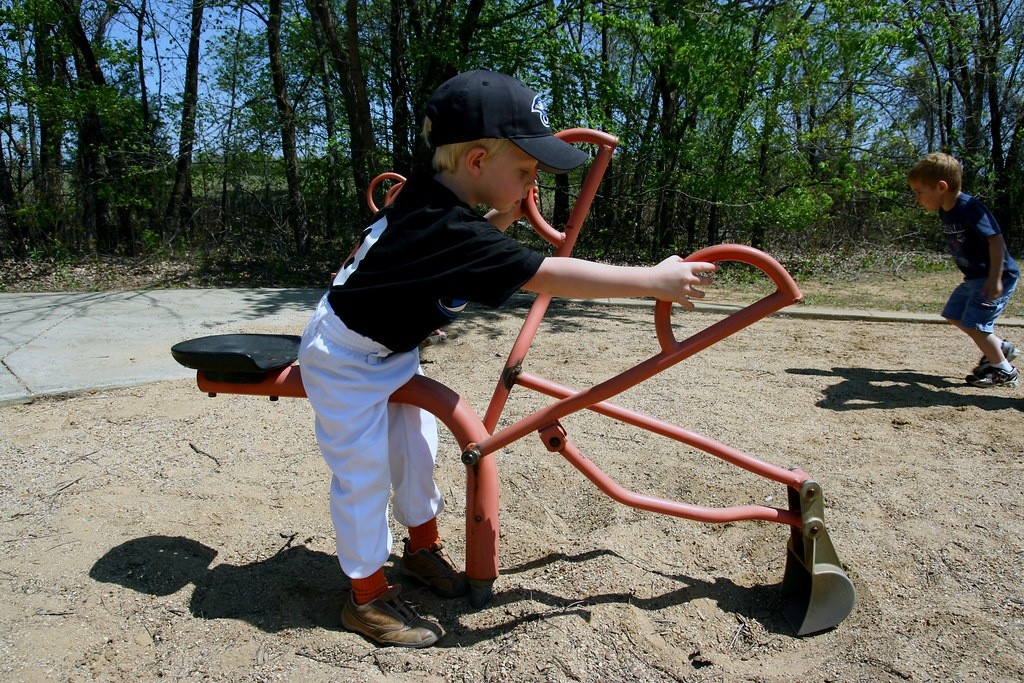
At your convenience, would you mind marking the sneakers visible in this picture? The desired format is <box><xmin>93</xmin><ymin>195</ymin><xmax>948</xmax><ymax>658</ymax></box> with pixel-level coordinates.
<box><xmin>398</xmin><ymin>536</ymin><xmax>464</xmax><ymax>599</ymax></box>
<box><xmin>979</xmin><ymin>339</ymin><xmax>1021</xmax><ymax>366</ymax></box>
<box><xmin>341</xmin><ymin>588</ymin><xmax>443</xmax><ymax>648</ymax></box>
<box><xmin>965</xmin><ymin>365</ymin><xmax>1019</xmax><ymax>388</ymax></box>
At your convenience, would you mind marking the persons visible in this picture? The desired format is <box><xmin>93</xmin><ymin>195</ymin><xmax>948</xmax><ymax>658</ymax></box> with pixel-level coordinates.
<box><xmin>298</xmin><ymin>72</ymin><xmax>717</xmax><ymax>646</ymax></box>
<box><xmin>907</xmin><ymin>153</ymin><xmax>1019</xmax><ymax>388</ymax></box>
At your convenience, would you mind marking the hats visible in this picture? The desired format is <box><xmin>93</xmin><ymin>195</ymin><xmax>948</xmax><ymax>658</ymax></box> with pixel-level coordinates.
<box><xmin>425</xmin><ymin>70</ymin><xmax>587</xmax><ymax>176</ymax></box>
